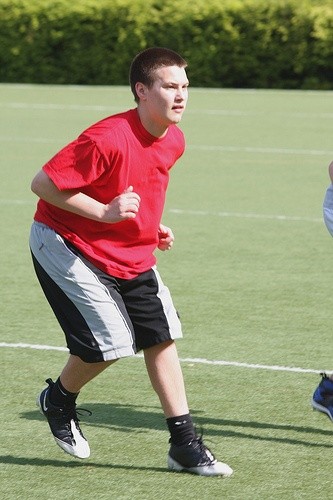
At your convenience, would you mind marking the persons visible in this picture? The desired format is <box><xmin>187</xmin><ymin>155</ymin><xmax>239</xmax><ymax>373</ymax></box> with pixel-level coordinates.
<box><xmin>28</xmin><ymin>48</ymin><xmax>234</xmax><ymax>477</ymax></box>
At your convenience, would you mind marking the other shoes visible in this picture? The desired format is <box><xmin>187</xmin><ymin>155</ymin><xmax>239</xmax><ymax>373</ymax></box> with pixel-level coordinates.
<box><xmin>311</xmin><ymin>373</ymin><xmax>333</xmax><ymax>423</ymax></box>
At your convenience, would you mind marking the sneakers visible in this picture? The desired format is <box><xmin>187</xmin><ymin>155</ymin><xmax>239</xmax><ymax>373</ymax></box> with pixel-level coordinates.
<box><xmin>36</xmin><ymin>378</ymin><xmax>92</xmax><ymax>458</ymax></box>
<box><xmin>168</xmin><ymin>427</ymin><xmax>233</xmax><ymax>476</ymax></box>
